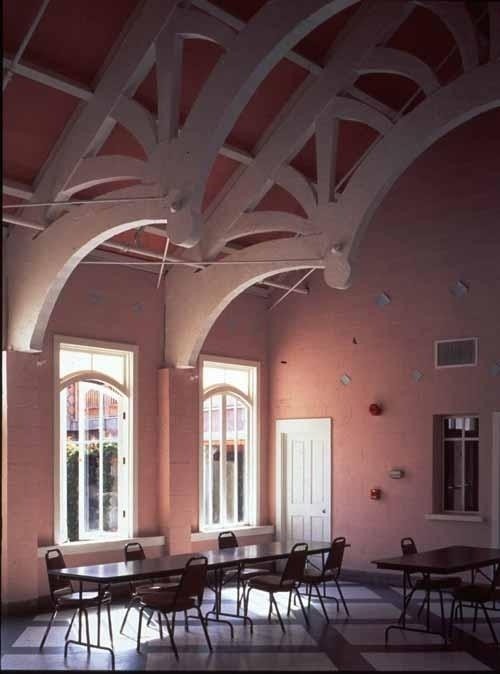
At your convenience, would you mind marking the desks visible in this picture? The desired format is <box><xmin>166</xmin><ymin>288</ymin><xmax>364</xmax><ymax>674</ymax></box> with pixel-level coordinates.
<box><xmin>47</xmin><ymin>539</ymin><xmax>351</xmax><ymax>671</ymax></box>
<box><xmin>371</xmin><ymin>545</ymin><xmax>500</xmax><ymax>649</ymax></box>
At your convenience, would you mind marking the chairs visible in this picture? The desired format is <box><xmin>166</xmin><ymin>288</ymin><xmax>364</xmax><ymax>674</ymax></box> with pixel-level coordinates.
<box><xmin>40</xmin><ymin>549</ymin><xmax>112</xmax><ymax>650</ymax></box>
<box><xmin>120</xmin><ymin>532</ymin><xmax>349</xmax><ymax>661</ymax></box>
<box><xmin>399</xmin><ymin>537</ymin><xmax>500</xmax><ymax>645</ymax></box>
